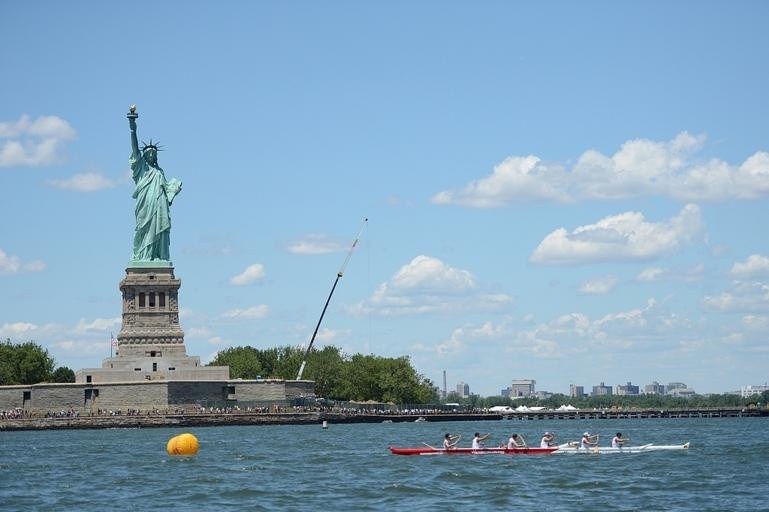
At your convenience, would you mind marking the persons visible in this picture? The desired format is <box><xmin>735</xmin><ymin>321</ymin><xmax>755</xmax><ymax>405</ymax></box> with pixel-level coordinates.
<box><xmin>507</xmin><ymin>432</ymin><xmax>525</xmax><ymax>449</ymax></box>
<box><xmin>0</xmin><ymin>401</ymin><xmax>494</xmax><ymax>420</ymax></box>
<box><xmin>611</xmin><ymin>432</ymin><xmax>625</xmax><ymax>448</ymax></box>
<box><xmin>443</xmin><ymin>432</ymin><xmax>461</xmax><ymax>449</ymax></box>
<box><xmin>582</xmin><ymin>431</ymin><xmax>597</xmax><ymax>447</ymax></box>
<box><xmin>471</xmin><ymin>431</ymin><xmax>490</xmax><ymax>448</ymax></box>
<box><xmin>125</xmin><ymin>110</ymin><xmax>180</xmax><ymax>261</ymax></box>
<box><xmin>539</xmin><ymin>431</ymin><xmax>554</xmax><ymax>447</ymax></box>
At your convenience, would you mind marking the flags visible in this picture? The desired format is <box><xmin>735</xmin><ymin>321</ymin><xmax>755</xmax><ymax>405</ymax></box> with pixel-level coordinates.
<box><xmin>111</xmin><ymin>334</ymin><xmax>118</xmax><ymax>349</ymax></box>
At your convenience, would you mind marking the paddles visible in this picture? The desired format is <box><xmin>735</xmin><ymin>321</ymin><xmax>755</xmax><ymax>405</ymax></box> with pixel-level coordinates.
<box><xmin>593</xmin><ymin>435</ymin><xmax>599</xmax><ymax>453</ymax></box>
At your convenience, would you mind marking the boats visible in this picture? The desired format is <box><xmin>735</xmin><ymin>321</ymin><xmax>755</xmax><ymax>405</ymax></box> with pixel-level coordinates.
<box><xmin>555</xmin><ymin>439</ymin><xmax>691</xmax><ymax>456</ymax></box>
<box><xmin>387</xmin><ymin>443</ymin><xmax>559</xmax><ymax>456</ymax></box>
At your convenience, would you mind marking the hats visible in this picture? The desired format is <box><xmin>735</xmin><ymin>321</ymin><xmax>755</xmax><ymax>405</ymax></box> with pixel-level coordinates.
<box><xmin>544</xmin><ymin>432</ymin><xmax>550</xmax><ymax>435</ymax></box>
<box><xmin>583</xmin><ymin>433</ymin><xmax>590</xmax><ymax>436</ymax></box>
